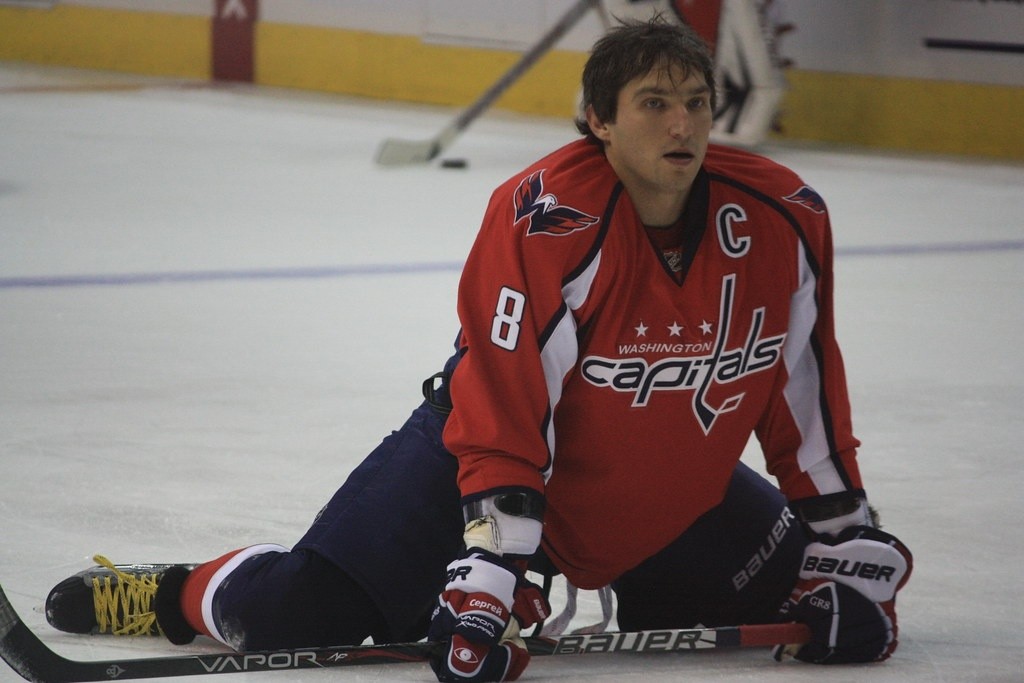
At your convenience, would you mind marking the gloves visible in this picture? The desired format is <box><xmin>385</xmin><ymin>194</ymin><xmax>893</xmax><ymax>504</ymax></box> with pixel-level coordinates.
<box><xmin>770</xmin><ymin>524</ymin><xmax>914</xmax><ymax>665</ymax></box>
<box><xmin>427</xmin><ymin>513</ymin><xmax>553</xmax><ymax>683</ymax></box>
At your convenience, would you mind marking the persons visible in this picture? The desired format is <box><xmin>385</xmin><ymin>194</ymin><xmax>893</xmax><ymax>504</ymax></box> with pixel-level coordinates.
<box><xmin>45</xmin><ymin>1</ymin><xmax>915</xmax><ymax>683</ymax></box>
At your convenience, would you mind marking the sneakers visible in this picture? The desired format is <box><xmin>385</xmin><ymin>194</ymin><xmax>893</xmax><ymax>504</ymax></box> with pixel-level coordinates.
<box><xmin>47</xmin><ymin>553</ymin><xmax>210</xmax><ymax>647</ymax></box>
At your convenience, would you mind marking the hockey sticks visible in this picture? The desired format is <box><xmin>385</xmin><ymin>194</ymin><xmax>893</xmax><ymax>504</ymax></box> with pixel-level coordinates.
<box><xmin>0</xmin><ymin>578</ymin><xmax>809</xmax><ymax>683</ymax></box>
<box><xmin>371</xmin><ymin>0</ymin><xmax>600</xmax><ymax>169</ymax></box>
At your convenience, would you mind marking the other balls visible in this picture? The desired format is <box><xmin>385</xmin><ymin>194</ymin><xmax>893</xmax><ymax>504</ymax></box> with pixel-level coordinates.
<box><xmin>440</xmin><ymin>159</ymin><xmax>466</xmax><ymax>169</ymax></box>
